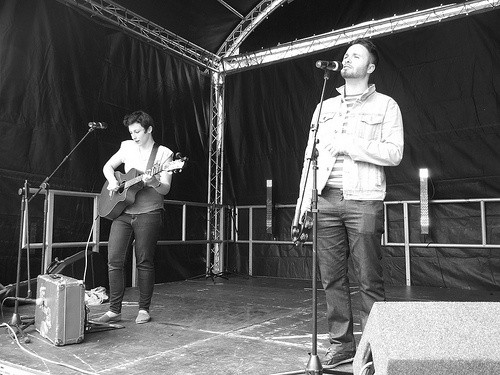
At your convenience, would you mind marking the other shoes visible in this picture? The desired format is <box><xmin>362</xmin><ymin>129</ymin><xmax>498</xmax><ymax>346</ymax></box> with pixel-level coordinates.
<box><xmin>135</xmin><ymin>311</ymin><xmax>151</xmax><ymax>324</ymax></box>
<box><xmin>319</xmin><ymin>350</ymin><xmax>355</xmax><ymax>368</ymax></box>
<box><xmin>94</xmin><ymin>312</ymin><xmax>122</xmax><ymax>322</ymax></box>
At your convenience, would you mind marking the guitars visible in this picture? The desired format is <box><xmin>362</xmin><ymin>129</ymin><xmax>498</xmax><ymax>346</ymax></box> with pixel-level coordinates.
<box><xmin>97</xmin><ymin>152</ymin><xmax>190</xmax><ymax>221</ymax></box>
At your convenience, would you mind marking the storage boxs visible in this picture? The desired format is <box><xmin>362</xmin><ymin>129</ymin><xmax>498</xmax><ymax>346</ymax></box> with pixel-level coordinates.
<box><xmin>35</xmin><ymin>275</ymin><xmax>86</xmax><ymax>346</ymax></box>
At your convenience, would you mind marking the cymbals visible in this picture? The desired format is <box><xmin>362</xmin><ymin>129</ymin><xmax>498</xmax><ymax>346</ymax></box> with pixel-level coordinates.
<box><xmin>291</xmin><ymin>210</ymin><xmax>314</xmax><ymax>248</ymax></box>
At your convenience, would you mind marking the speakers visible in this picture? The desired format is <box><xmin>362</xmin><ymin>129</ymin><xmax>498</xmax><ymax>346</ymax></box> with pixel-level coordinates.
<box><xmin>352</xmin><ymin>302</ymin><xmax>500</xmax><ymax>375</ymax></box>
<box><xmin>35</xmin><ymin>273</ymin><xmax>86</xmax><ymax>346</ymax></box>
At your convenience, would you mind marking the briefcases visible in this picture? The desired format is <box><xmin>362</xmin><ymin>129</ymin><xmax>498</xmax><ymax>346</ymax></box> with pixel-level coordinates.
<box><xmin>35</xmin><ymin>273</ymin><xmax>84</xmax><ymax>345</ymax></box>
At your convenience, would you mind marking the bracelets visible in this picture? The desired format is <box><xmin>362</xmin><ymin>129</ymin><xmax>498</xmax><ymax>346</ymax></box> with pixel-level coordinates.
<box><xmin>153</xmin><ymin>182</ymin><xmax>161</xmax><ymax>188</ymax></box>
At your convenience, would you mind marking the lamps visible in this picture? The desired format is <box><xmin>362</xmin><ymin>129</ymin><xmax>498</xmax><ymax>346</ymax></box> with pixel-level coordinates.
<box><xmin>265</xmin><ymin>179</ymin><xmax>272</xmax><ymax>234</ymax></box>
<box><xmin>418</xmin><ymin>167</ymin><xmax>429</xmax><ymax>235</ymax></box>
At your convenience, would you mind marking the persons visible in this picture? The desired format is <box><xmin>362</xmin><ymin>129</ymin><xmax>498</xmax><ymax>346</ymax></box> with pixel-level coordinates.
<box><xmin>87</xmin><ymin>111</ymin><xmax>175</xmax><ymax>325</ymax></box>
<box><xmin>288</xmin><ymin>38</ymin><xmax>405</xmax><ymax>375</ymax></box>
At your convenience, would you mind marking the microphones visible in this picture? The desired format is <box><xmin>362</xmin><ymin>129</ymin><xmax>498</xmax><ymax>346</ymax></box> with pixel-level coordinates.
<box><xmin>316</xmin><ymin>60</ymin><xmax>344</xmax><ymax>71</ymax></box>
<box><xmin>8</xmin><ymin>296</ymin><xmax>44</xmax><ymax>307</ymax></box>
<box><xmin>89</xmin><ymin>121</ymin><xmax>108</xmax><ymax>128</ymax></box>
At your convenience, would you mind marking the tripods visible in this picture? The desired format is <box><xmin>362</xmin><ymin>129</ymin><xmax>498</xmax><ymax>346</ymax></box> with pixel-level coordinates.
<box><xmin>185</xmin><ymin>206</ymin><xmax>249</xmax><ymax>284</ymax></box>
<box><xmin>0</xmin><ymin>127</ymin><xmax>96</xmax><ymax>344</ymax></box>
<box><xmin>275</xmin><ymin>68</ymin><xmax>355</xmax><ymax>375</ymax></box>
